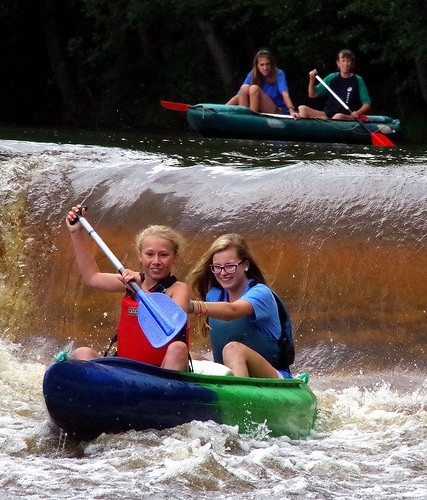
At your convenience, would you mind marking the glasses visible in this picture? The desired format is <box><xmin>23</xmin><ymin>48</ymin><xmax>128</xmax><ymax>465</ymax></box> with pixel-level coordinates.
<box><xmin>209</xmin><ymin>259</ymin><xmax>242</xmax><ymax>274</ymax></box>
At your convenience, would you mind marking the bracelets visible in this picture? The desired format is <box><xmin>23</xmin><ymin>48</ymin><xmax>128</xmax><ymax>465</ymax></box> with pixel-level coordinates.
<box><xmin>204</xmin><ymin>302</ymin><xmax>209</xmax><ymax>316</ymax></box>
<box><xmin>197</xmin><ymin>300</ymin><xmax>202</xmax><ymax>318</ymax></box>
<box><xmin>189</xmin><ymin>301</ymin><xmax>194</xmax><ymax>313</ymax></box>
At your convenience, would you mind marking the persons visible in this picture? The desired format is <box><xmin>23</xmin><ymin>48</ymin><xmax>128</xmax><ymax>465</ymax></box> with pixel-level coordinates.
<box><xmin>225</xmin><ymin>49</ymin><xmax>301</xmax><ymax>121</ymax></box>
<box><xmin>65</xmin><ymin>203</ymin><xmax>190</xmax><ymax>371</ymax></box>
<box><xmin>186</xmin><ymin>231</ymin><xmax>293</xmax><ymax>379</ymax></box>
<box><xmin>298</xmin><ymin>48</ymin><xmax>372</xmax><ymax>120</ymax></box>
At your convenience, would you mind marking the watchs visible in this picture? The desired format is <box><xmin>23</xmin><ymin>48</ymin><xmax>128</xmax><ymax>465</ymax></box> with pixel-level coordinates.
<box><xmin>290</xmin><ymin>106</ymin><xmax>295</xmax><ymax>110</ymax></box>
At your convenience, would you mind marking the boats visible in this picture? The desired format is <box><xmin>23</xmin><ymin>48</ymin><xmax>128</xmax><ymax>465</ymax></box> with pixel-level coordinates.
<box><xmin>42</xmin><ymin>349</ymin><xmax>319</xmax><ymax>448</ymax></box>
<box><xmin>186</xmin><ymin>103</ymin><xmax>401</xmax><ymax>146</ymax></box>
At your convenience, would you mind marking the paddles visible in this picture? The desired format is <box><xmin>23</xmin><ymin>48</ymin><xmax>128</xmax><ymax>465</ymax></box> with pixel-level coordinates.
<box><xmin>313</xmin><ymin>73</ymin><xmax>394</xmax><ymax>146</ymax></box>
<box><xmin>159</xmin><ymin>100</ymin><xmax>294</xmax><ymax>118</ymax></box>
<box><xmin>68</xmin><ymin>205</ymin><xmax>190</xmax><ymax>350</ymax></box>
